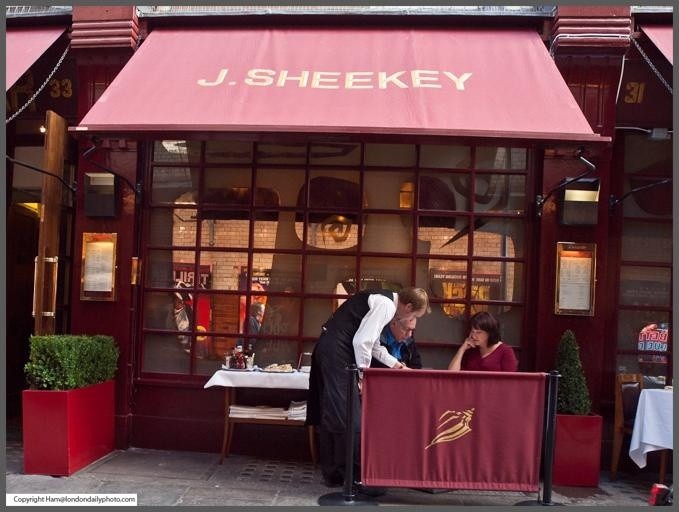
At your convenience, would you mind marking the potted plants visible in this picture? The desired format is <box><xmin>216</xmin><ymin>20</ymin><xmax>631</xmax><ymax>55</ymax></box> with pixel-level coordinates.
<box><xmin>21</xmin><ymin>334</ymin><xmax>121</xmax><ymax>476</ymax></box>
<box><xmin>549</xmin><ymin>330</ymin><xmax>603</xmax><ymax>487</ymax></box>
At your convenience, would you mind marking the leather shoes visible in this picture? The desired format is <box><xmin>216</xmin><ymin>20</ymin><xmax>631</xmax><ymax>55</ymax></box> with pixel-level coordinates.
<box><xmin>324</xmin><ymin>476</ymin><xmax>387</xmax><ymax>497</ymax></box>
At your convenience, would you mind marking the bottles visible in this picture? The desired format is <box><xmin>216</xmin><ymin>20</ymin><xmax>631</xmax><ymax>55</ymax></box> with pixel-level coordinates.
<box><xmin>224</xmin><ymin>343</ymin><xmax>254</xmax><ymax>369</ymax></box>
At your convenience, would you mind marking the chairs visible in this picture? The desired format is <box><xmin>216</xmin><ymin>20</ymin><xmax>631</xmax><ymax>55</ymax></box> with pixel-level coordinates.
<box><xmin>610</xmin><ymin>372</ymin><xmax>673</xmax><ymax>484</ymax></box>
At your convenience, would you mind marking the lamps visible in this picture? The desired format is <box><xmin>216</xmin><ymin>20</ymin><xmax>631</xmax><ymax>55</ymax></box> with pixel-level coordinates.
<box><xmin>399</xmin><ymin>183</ymin><xmax>413</xmax><ymax>208</ymax></box>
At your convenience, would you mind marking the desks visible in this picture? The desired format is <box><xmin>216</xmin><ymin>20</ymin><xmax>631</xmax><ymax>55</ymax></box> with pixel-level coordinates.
<box><xmin>204</xmin><ymin>369</ymin><xmax>317</xmax><ymax>469</ymax></box>
<box><xmin>628</xmin><ymin>389</ymin><xmax>673</xmax><ymax>469</ymax></box>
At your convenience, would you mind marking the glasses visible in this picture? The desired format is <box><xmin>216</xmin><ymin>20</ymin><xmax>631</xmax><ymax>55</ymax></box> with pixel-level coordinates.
<box><xmin>399</xmin><ymin>321</ymin><xmax>416</xmax><ymax>334</ymax></box>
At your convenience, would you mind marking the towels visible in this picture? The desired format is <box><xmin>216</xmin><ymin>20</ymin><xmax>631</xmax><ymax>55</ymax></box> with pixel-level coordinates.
<box><xmin>229</xmin><ymin>401</ymin><xmax>307</xmax><ymax>422</ymax></box>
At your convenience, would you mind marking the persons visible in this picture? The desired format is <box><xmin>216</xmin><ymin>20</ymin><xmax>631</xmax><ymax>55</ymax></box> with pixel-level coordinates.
<box><xmin>447</xmin><ymin>311</ymin><xmax>521</xmax><ymax>371</ymax></box>
<box><xmin>238</xmin><ymin>302</ymin><xmax>267</xmax><ymax>350</ymax></box>
<box><xmin>367</xmin><ymin>316</ymin><xmax>423</xmax><ymax>369</ymax></box>
<box><xmin>306</xmin><ymin>284</ymin><xmax>429</xmax><ymax>491</ymax></box>
